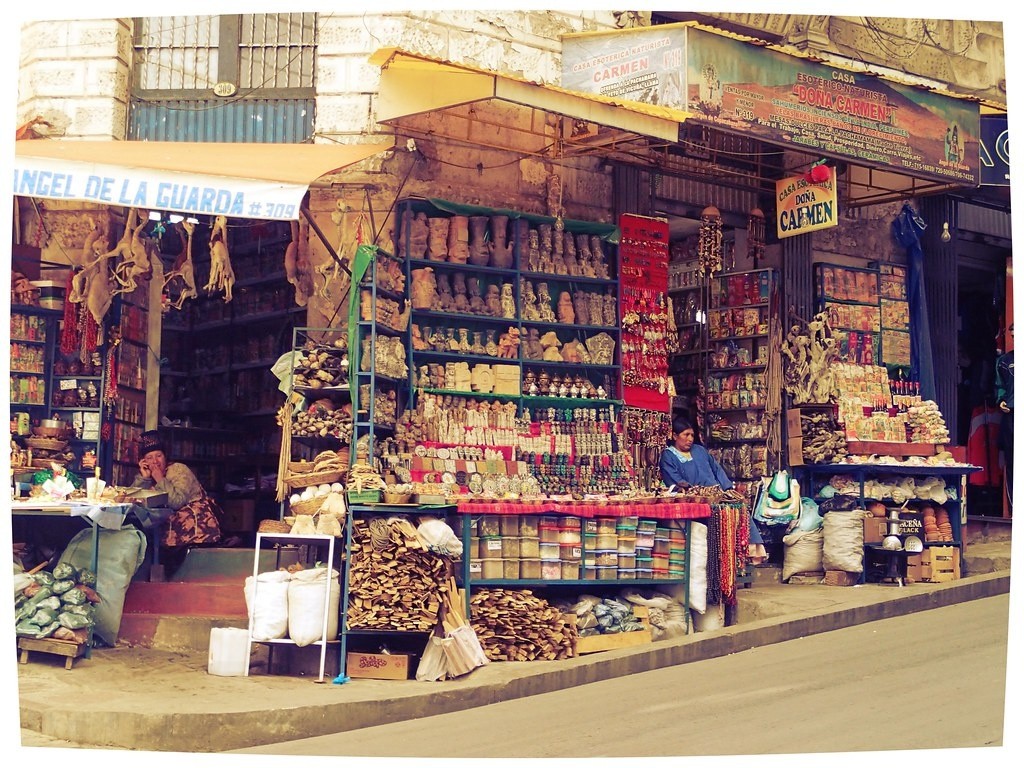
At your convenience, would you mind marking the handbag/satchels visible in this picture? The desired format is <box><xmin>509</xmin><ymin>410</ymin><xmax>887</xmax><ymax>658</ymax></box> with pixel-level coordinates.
<box><xmin>752</xmin><ymin>470</ymin><xmax>803</xmax><ymax>527</ymax></box>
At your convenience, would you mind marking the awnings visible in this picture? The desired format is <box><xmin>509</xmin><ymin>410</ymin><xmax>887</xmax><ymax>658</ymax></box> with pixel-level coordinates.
<box><xmin>368</xmin><ymin>21</ymin><xmax>986</xmax><ymax>188</ymax></box>
<box><xmin>15</xmin><ymin>141</ymin><xmax>393</xmax><ymax>222</ymax></box>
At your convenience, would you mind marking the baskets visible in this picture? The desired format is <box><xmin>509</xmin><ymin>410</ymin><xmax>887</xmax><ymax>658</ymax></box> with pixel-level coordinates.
<box><xmin>25</xmin><ymin>439</ymin><xmax>68</xmax><ymax>451</ymax></box>
<box><xmin>282</xmin><ymin>462</ymin><xmax>348</xmax><ymax>488</ymax></box>
<box><xmin>33</xmin><ymin>427</ymin><xmax>76</xmax><ymax>436</ymax></box>
<box><xmin>259</xmin><ymin>520</ymin><xmax>291</xmax><ymax>534</ymax></box>
<box><xmin>290</xmin><ymin>489</ymin><xmax>345</xmax><ymax>515</ymax></box>
<box><xmin>11</xmin><ymin>459</ymin><xmax>68</xmax><ymax>474</ymax></box>
<box><xmin>345</xmin><ymin>488</ymin><xmax>411</xmax><ymax>504</ymax></box>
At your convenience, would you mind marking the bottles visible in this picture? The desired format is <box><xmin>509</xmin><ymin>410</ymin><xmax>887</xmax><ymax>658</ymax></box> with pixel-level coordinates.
<box><xmin>870</xmin><ymin>369</ymin><xmax>923</xmax><ymax>425</ymax></box>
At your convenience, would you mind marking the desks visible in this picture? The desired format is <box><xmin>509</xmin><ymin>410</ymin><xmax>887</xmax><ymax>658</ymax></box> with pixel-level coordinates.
<box><xmin>11</xmin><ymin>503</ymin><xmax>160</xmax><ymax>612</ymax></box>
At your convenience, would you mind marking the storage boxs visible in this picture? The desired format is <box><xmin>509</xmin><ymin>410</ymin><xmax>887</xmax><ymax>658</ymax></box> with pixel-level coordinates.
<box><xmin>906</xmin><ymin>547</ymin><xmax>961</xmax><ymax>583</ymax></box>
<box><xmin>788</xmin><ymin>436</ymin><xmax>840</xmax><ymax>466</ymax></box>
<box><xmin>455</xmin><ymin>516</ymin><xmax>687</xmax><ymax>580</ymax></box>
<box><xmin>346</xmin><ymin>653</ymin><xmax>410</xmax><ymax>680</ymax></box>
<box><xmin>865</xmin><ymin>518</ymin><xmax>888</xmax><ymax>544</ymax></box>
<box><xmin>787</xmin><ymin>408</ymin><xmax>834</xmax><ymax>438</ymax></box>
<box><xmin>224</xmin><ymin>499</ymin><xmax>254</xmax><ymax>532</ymax></box>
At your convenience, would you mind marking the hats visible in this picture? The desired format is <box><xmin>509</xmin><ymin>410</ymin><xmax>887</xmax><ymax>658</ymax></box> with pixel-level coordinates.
<box><xmin>138</xmin><ymin>430</ymin><xmax>165</xmax><ymax>455</ymax></box>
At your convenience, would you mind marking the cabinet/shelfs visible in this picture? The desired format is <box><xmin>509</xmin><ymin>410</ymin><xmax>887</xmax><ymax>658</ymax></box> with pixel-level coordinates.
<box><xmin>665</xmin><ymin>255</ymin><xmax>982</xmax><ymax>586</ymax></box>
<box><xmin>279</xmin><ymin>202</ymin><xmax>744</xmax><ymax>638</ymax></box>
<box><xmin>244</xmin><ymin>533</ymin><xmax>344</xmax><ymax>681</ymax></box>
<box><xmin>9</xmin><ymin>221</ymin><xmax>314</xmax><ymax>539</ymax></box>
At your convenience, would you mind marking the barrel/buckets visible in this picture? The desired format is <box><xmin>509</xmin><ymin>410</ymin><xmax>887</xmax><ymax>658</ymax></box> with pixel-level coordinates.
<box><xmin>208</xmin><ymin>628</ymin><xmax>249</xmax><ymax>676</ymax></box>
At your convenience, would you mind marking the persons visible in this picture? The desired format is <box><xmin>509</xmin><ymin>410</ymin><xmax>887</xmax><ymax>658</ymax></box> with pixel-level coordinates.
<box><xmin>996</xmin><ymin>323</ymin><xmax>1014</xmax><ymax>519</ymax></box>
<box><xmin>130</xmin><ymin>430</ymin><xmax>225</xmax><ymax>581</ymax></box>
<box><xmin>661</xmin><ymin>418</ymin><xmax>768</xmax><ymax>565</ymax></box>
